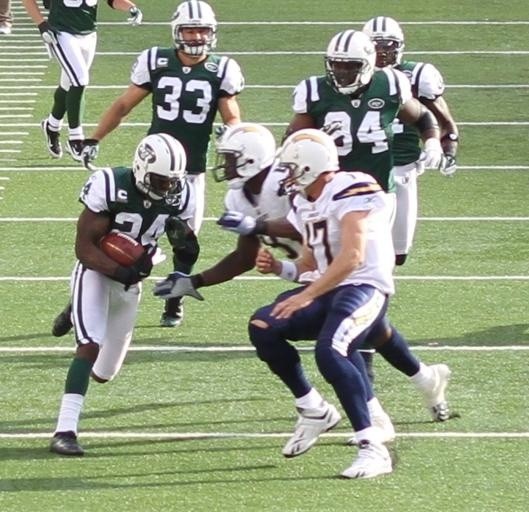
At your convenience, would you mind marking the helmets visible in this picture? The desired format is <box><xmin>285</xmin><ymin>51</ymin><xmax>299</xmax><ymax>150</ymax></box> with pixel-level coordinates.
<box><xmin>211</xmin><ymin>121</ymin><xmax>275</xmax><ymax>190</ymax></box>
<box><xmin>323</xmin><ymin>29</ymin><xmax>376</xmax><ymax>95</ymax></box>
<box><xmin>131</xmin><ymin>132</ymin><xmax>187</xmax><ymax>203</ymax></box>
<box><xmin>170</xmin><ymin>0</ymin><xmax>218</xmax><ymax>58</ymax></box>
<box><xmin>362</xmin><ymin>15</ymin><xmax>405</xmax><ymax>69</ymax></box>
<box><xmin>276</xmin><ymin>128</ymin><xmax>340</xmax><ymax>198</ymax></box>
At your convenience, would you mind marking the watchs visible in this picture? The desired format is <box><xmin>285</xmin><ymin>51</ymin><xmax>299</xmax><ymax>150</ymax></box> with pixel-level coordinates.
<box><xmin>441</xmin><ymin>133</ymin><xmax>459</xmax><ymax>143</ymax></box>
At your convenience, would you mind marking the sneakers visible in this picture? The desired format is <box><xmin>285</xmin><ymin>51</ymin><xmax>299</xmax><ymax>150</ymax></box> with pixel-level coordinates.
<box><xmin>159</xmin><ymin>297</ymin><xmax>184</xmax><ymax>327</ymax></box>
<box><xmin>424</xmin><ymin>364</ymin><xmax>452</xmax><ymax>422</ymax></box>
<box><xmin>281</xmin><ymin>404</ymin><xmax>342</xmax><ymax>457</ymax></box>
<box><xmin>51</xmin><ymin>296</ymin><xmax>72</xmax><ymax>338</ymax></box>
<box><xmin>338</xmin><ymin>440</ymin><xmax>392</xmax><ymax>480</ymax></box>
<box><xmin>0</xmin><ymin>21</ymin><xmax>11</xmax><ymax>34</ymax></box>
<box><xmin>41</xmin><ymin>119</ymin><xmax>63</xmax><ymax>157</ymax></box>
<box><xmin>48</xmin><ymin>432</ymin><xmax>85</xmax><ymax>455</ymax></box>
<box><xmin>64</xmin><ymin>137</ymin><xmax>85</xmax><ymax>161</ymax></box>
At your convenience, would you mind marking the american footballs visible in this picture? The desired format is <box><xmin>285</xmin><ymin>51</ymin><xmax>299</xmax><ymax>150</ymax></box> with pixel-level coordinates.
<box><xmin>97</xmin><ymin>233</ymin><xmax>144</xmax><ymax>266</ymax></box>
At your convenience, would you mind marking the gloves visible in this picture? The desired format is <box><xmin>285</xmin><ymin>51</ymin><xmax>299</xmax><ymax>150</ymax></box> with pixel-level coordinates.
<box><xmin>37</xmin><ymin>21</ymin><xmax>62</xmax><ymax>45</ymax></box>
<box><xmin>216</xmin><ymin>210</ymin><xmax>267</xmax><ymax>239</ymax></box>
<box><xmin>81</xmin><ymin>138</ymin><xmax>100</xmax><ymax>171</ymax></box>
<box><xmin>127</xmin><ymin>7</ymin><xmax>143</xmax><ymax>28</ymax></box>
<box><xmin>152</xmin><ymin>271</ymin><xmax>204</xmax><ymax>303</ymax></box>
<box><xmin>122</xmin><ymin>253</ymin><xmax>153</xmax><ymax>285</ymax></box>
<box><xmin>163</xmin><ymin>216</ymin><xmax>186</xmax><ymax>249</ymax></box>
<box><xmin>418</xmin><ymin>138</ymin><xmax>443</xmax><ymax>171</ymax></box>
<box><xmin>214</xmin><ymin>124</ymin><xmax>228</xmax><ymax>139</ymax></box>
<box><xmin>439</xmin><ymin>154</ymin><xmax>458</xmax><ymax>176</ymax></box>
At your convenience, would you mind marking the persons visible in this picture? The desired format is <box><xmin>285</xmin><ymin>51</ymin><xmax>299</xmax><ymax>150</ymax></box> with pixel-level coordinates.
<box><xmin>21</xmin><ymin>0</ymin><xmax>143</xmax><ymax>162</ymax></box>
<box><xmin>360</xmin><ymin>16</ymin><xmax>459</xmax><ymax>266</ymax></box>
<box><xmin>79</xmin><ymin>0</ymin><xmax>246</xmax><ymax>329</ymax></box>
<box><xmin>0</xmin><ymin>0</ymin><xmax>14</xmax><ymax>35</ymax></box>
<box><xmin>280</xmin><ymin>29</ymin><xmax>447</xmax><ymax>194</ymax></box>
<box><xmin>247</xmin><ymin>128</ymin><xmax>397</xmax><ymax>480</ymax></box>
<box><xmin>150</xmin><ymin>121</ymin><xmax>452</xmax><ymax>446</ymax></box>
<box><xmin>49</xmin><ymin>131</ymin><xmax>196</xmax><ymax>456</ymax></box>
